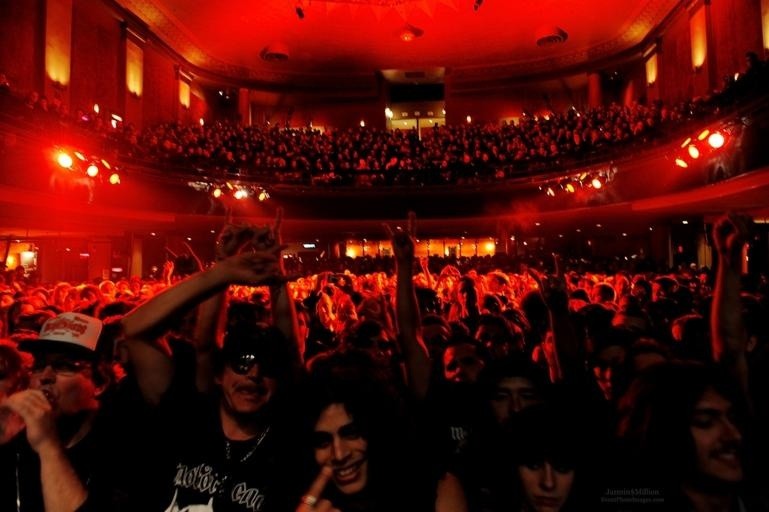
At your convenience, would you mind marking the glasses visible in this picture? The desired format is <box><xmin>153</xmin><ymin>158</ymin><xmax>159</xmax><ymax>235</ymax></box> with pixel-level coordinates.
<box><xmin>229</xmin><ymin>350</ymin><xmax>279</xmax><ymax>380</ymax></box>
<box><xmin>31</xmin><ymin>358</ymin><xmax>93</xmax><ymax>374</ymax></box>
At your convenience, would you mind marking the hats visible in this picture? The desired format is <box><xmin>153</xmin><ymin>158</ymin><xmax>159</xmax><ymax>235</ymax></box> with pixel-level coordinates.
<box><xmin>18</xmin><ymin>313</ymin><xmax>113</xmax><ymax>366</ymax></box>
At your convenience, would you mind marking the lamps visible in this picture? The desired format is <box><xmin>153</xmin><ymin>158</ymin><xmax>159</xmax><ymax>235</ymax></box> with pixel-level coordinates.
<box><xmin>54</xmin><ymin>150</ymin><xmax>120</xmax><ymax>189</ymax></box>
<box><xmin>669</xmin><ymin>122</ymin><xmax>736</xmax><ymax>172</ymax></box>
<box><xmin>199</xmin><ymin>182</ymin><xmax>271</xmax><ymax>204</ymax></box>
<box><xmin>540</xmin><ymin>166</ymin><xmax>615</xmax><ymax>198</ymax></box>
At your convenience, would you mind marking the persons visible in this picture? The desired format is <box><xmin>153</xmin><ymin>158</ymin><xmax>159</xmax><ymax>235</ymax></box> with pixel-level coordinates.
<box><xmin>1</xmin><ymin>51</ymin><xmax>768</xmax><ymax>183</ymax></box>
<box><xmin>2</xmin><ymin>207</ymin><xmax>769</xmax><ymax>510</ymax></box>
